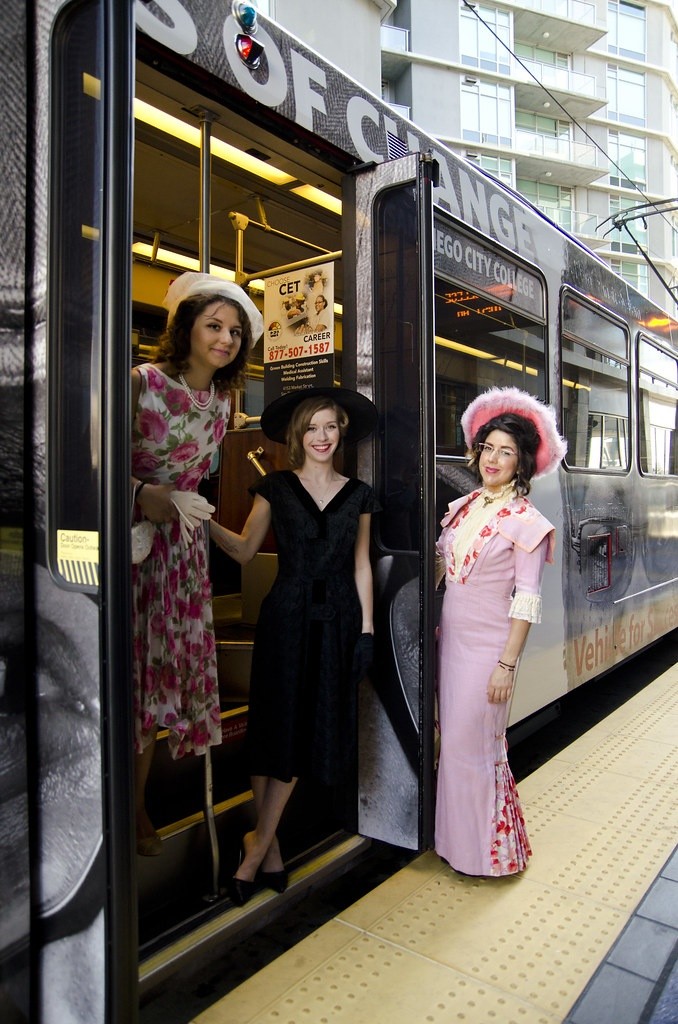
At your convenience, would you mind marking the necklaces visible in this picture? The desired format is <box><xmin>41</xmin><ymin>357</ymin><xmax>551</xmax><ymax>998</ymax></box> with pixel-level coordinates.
<box><xmin>301</xmin><ymin>469</ymin><xmax>334</xmax><ymax>505</ymax></box>
<box><xmin>178</xmin><ymin>373</ymin><xmax>215</xmax><ymax>410</ymax></box>
<box><xmin>479</xmin><ymin>483</ymin><xmax>514</xmax><ymax>507</ymax></box>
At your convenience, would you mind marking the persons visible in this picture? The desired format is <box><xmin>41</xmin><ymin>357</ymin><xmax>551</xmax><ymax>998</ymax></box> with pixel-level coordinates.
<box><xmin>431</xmin><ymin>386</ymin><xmax>569</xmax><ymax>879</ymax></box>
<box><xmin>130</xmin><ymin>274</ymin><xmax>267</xmax><ymax>859</ymax></box>
<box><xmin>281</xmin><ymin>272</ymin><xmax>331</xmax><ymax>334</ymax></box>
<box><xmin>197</xmin><ymin>387</ymin><xmax>387</xmax><ymax>909</ymax></box>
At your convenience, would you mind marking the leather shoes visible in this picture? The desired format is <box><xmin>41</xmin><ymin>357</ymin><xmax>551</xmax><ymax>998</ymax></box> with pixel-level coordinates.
<box><xmin>260</xmin><ymin>866</ymin><xmax>289</xmax><ymax>893</ymax></box>
<box><xmin>230</xmin><ymin>846</ymin><xmax>258</xmax><ymax>906</ymax></box>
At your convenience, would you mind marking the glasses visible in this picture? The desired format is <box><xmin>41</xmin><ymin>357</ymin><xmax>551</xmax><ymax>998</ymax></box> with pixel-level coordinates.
<box><xmin>478</xmin><ymin>442</ymin><xmax>521</xmax><ymax>463</ymax></box>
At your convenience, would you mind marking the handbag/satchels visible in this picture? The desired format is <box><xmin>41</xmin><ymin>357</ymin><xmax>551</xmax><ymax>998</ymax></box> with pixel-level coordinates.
<box><xmin>131</xmin><ymin>518</ymin><xmax>156</xmax><ymax>563</ymax></box>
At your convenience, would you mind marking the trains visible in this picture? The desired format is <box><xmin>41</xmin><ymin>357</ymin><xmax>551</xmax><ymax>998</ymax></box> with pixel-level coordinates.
<box><xmin>1</xmin><ymin>0</ymin><xmax>678</xmax><ymax>1022</ymax></box>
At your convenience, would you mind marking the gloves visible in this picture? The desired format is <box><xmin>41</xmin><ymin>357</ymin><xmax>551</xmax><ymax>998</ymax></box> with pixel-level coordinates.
<box><xmin>171</xmin><ymin>489</ymin><xmax>215</xmax><ymax>544</ymax></box>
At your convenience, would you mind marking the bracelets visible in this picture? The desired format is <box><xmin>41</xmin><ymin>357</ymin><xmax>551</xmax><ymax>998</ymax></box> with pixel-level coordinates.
<box><xmin>134</xmin><ymin>481</ymin><xmax>146</xmax><ymax>498</ymax></box>
<box><xmin>498</xmin><ymin>664</ymin><xmax>514</xmax><ymax>671</ymax></box>
<box><xmin>498</xmin><ymin>660</ymin><xmax>515</xmax><ymax>668</ymax></box>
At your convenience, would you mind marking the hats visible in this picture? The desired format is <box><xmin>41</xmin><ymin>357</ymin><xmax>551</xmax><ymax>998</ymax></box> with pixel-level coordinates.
<box><xmin>257</xmin><ymin>387</ymin><xmax>378</xmax><ymax>448</ymax></box>
<box><xmin>162</xmin><ymin>274</ymin><xmax>266</xmax><ymax>348</ymax></box>
<box><xmin>460</xmin><ymin>388</ymin><xmax>567</xmax><ymax>476</ymax></box>
